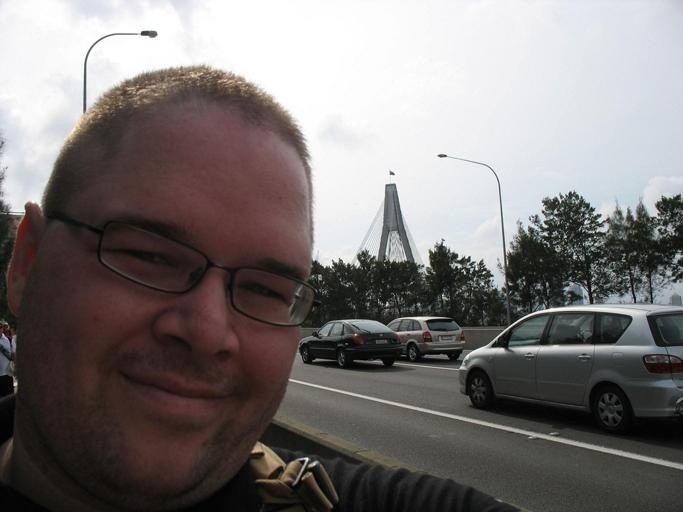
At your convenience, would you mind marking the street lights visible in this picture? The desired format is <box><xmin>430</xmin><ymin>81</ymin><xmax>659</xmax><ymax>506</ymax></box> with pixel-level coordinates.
<box><xmin>437</xmin><ymin>153</ymin><xmax>513</xmax><ymax>326</ymax></box>
<box><xmin>82</xmin><ymin>29</ymin><xmax>159</xmax><ymax>114</ymax></box>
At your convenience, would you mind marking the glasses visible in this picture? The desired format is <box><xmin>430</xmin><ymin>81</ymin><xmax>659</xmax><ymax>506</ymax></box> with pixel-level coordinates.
<box><xmin>46</xmin><ymin>211</ymin><xmax>325</xmax><ymax>328</ymax></box>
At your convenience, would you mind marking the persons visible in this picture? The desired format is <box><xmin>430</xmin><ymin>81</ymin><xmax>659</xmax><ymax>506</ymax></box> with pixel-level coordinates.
<box><xmin>0</xmin><ymin>320</ymin><xmax>14</xmax><ymax>399</ymax></box>
<box><xmin>2</xmin><ymin>323</ymin><xmax>11</xmax><ymax>337</ymax></box>
<box><xmin>0</xmin><ymin>64</ymin><xmax>524</xmax><ymax>511</ymax></box>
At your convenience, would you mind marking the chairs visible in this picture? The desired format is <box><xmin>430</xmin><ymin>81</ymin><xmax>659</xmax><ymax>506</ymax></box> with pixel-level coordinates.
<box><xmin>551</xmin><ymin>325</ymin><xmax>577</xmax><ymax>345</ymax></box>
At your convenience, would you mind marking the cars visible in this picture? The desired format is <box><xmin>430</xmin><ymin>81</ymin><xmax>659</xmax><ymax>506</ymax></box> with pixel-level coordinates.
<box><xmin>298</xmin><ymin>316</ymin><xmax>465</xmax><ymax>369</ymax></box>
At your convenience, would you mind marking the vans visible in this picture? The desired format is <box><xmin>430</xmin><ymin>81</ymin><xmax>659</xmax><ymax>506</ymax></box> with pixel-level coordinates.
<box><xmin>456</xmin><ymin>303</ymin><xmax>683</xmax><ymax>435</ymax></box>
